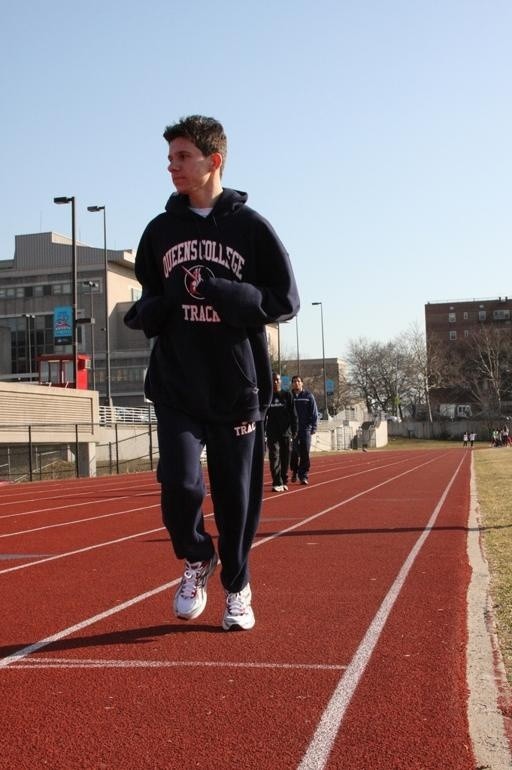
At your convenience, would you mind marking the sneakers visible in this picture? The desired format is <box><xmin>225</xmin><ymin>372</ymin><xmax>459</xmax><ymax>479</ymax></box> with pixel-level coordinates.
<box><xmin>271</xmin><ymin>473</ymin><xmax>310</xmax><ymax>493</ymax></box>
<box><xmin>173</xmin><ymin>552</ymin><xmax>219</xmax><ymax>622</ymax></box>
<box><xmin>221</xmin><ymin>580</ymin><xmax>256</xmax><ymax>632</ymax></box>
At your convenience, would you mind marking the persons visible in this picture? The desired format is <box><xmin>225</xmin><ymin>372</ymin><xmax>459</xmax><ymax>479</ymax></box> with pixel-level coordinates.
<box><xmin>263</xmin><ymin>371</ymin><xmax>300</xmax><ymax>493</ymax></box>
<box><xmin>289</xmin><ymin>374</ymin><xmax>318</xmax><ymax>487</ymax></box>
<box><xmin>487</xmin><ymin>424</ymin><xmax>512</xmax><ymax>448</ymax></box>
<box><xmin>463</xmin><ymin>431</ymin><xmax>470</xmax><ymax>448</ymax></box>
<box><xmin>121</xmin><ymin>115</ymin><xmax>302</xmax><ymax>633</ymax></box>
<box><xmin>468</xmin><ymin>431</ymin><xmax>478</xmax><ymax>448</ymax></box>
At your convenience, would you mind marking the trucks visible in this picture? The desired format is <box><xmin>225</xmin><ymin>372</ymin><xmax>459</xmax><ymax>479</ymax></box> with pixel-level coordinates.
<box><xmin>440</xmin><ymin>404</ymin><xmax>472</xmax><ymax>418</ymax></box>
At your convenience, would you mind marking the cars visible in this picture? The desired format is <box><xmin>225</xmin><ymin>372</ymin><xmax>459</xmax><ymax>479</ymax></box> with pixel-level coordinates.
<box><xmin>387</xmin><ymin>416</ymin><xmax>402</xmax><ymax>423</ymax></box>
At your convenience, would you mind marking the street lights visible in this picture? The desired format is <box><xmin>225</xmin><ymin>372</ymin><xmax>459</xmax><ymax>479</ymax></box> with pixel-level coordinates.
<box><xmin>87</xmin><ymin>205</ymin><xmax>114</xmax><ymax>426</ymax></box>
<box><xmin>312</xmin><ymin>302</ymin><xmax>327</xmax><ymax>409</ymax></box>
<box><xmin>22</xmin><ymin>314</ymin><xmax>35</xmax><ymax>382</ymax></box>
<box><xmin>83</xmin><ymin>281</ymin><xmax>99</xmax><ymax>391</ymax></box>
<box><xmin>54</xmin><ymin>196</ymin><xmax>78</xmax><ymax>390</ymax></box>
